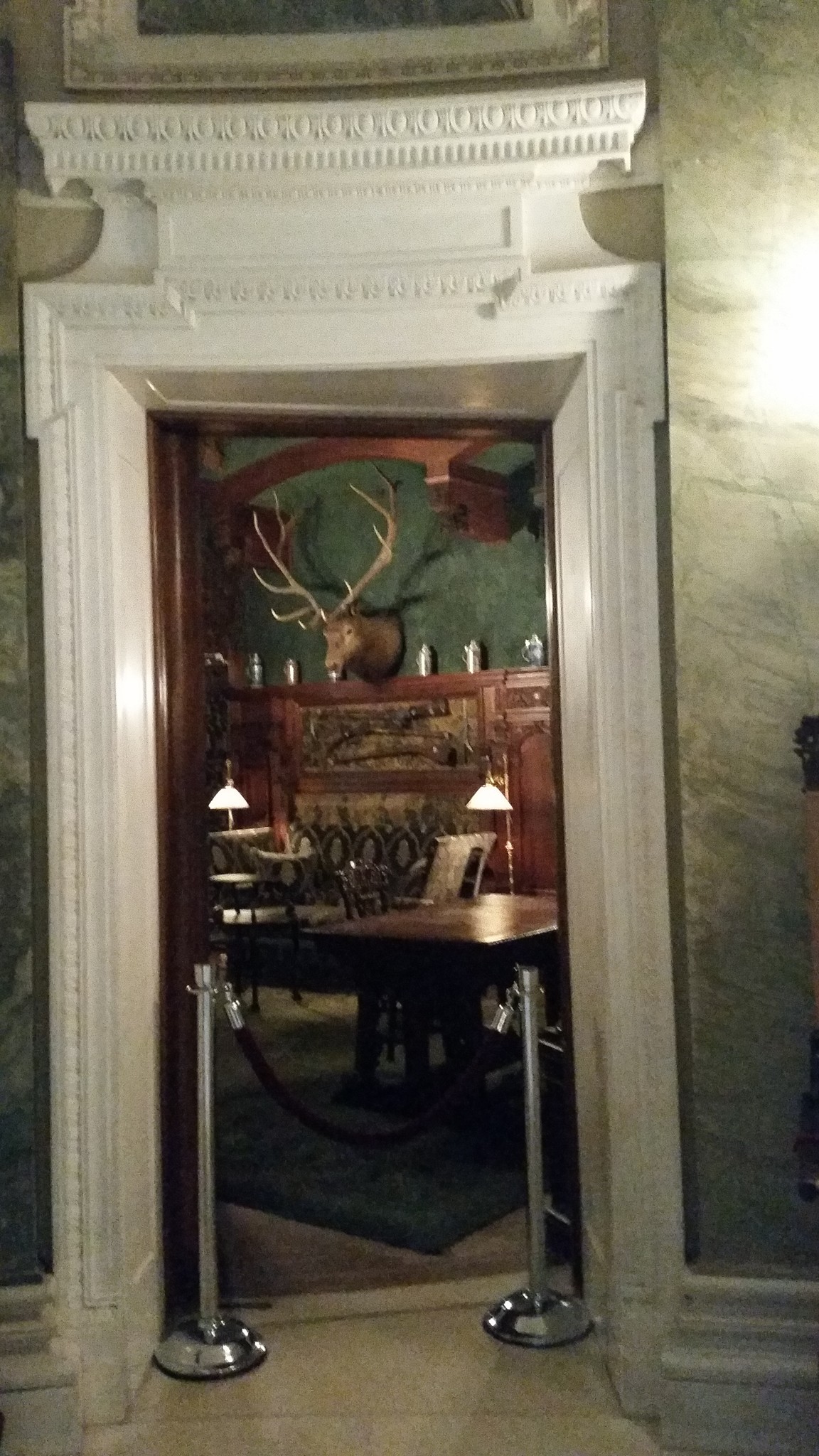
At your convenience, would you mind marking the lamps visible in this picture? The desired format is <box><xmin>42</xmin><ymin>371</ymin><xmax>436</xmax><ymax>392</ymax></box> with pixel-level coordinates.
<box><xmin>466</xmin><ymin>752</ymin><xmax>519</xmax><ymax>1052</ymax></box>
<box><xmin>209</xmin><ymin>753</ymin><xmax>253</xmax><ymax>995</ymax></box>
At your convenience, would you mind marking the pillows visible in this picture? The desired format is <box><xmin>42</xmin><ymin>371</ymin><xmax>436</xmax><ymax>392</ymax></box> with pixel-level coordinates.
<box><xmin>402</xmin><ymin>846</ymin><xmax>431</xmax><ymax>906</ymax></box>
<box><xmin>247</xmin><ymin>844</ymin><xmax>320</xmax><ymax>908</ymax></box>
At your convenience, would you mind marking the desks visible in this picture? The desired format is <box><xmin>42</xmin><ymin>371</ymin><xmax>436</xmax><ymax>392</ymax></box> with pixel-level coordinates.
<box><xmin>300</xmin><ymin>886</ymin><xmax>567</xmax><ymax>1163</ymax></box>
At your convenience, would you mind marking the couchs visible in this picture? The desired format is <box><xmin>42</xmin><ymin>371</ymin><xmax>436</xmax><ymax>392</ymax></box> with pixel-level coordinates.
<box><xmin>207</xmin><ymin>824</ymin><xmax>493</xmax><ymax>993</ymax></box>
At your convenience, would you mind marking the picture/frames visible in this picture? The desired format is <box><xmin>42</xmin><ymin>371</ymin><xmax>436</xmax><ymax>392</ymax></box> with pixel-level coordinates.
<box><xmin>58</xmin><ymin>1</ymin><xmax>613</xmax><ymax>92</ymax></box>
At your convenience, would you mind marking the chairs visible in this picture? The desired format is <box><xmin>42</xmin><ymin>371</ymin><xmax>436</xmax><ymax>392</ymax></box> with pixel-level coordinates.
<box><xmin>414</xmin><ymin>831</ymin><xmax>502</xmax><ymax>907</ymax></box>
<box><xmin>212</xmin><ymin>877</ymin><xmax>304</xmax><ymax>1012</ymax></box>
<box><xmin>333</xmin><ymin>854</ymin><xmax>464</xmax><ymax>1085</ymax></box>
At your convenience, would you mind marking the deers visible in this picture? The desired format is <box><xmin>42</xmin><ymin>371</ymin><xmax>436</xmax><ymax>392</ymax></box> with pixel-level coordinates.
<box><xmin>251</xmin><ymin>459</ymin><xmax>407</xmax><ymax>684</ymax></box>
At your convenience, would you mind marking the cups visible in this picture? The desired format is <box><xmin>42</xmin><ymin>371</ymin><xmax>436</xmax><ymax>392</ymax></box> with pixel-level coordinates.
<box><xmin>247</xmin><ymin>653</ymin><xmax>263</xmax><ymax>689</ymax></box>
<box><xmin>284</xmin><ymin>659</ymin><xmax>298</xmax><ymax>686</ymax></box>
<box><xmin>520</xmin><ymin>634</ymin><xmax>546</xmax><ymax>666</ymax></box>
<box><xmin>414</xmin><ymin>643</ymin><xmax>434</xmax><ymax>676</ymax></box>
<box><xmin>461</xmin><ymin>640</ymin><xmax>482</xmax><ymax>674</ymax></box>
